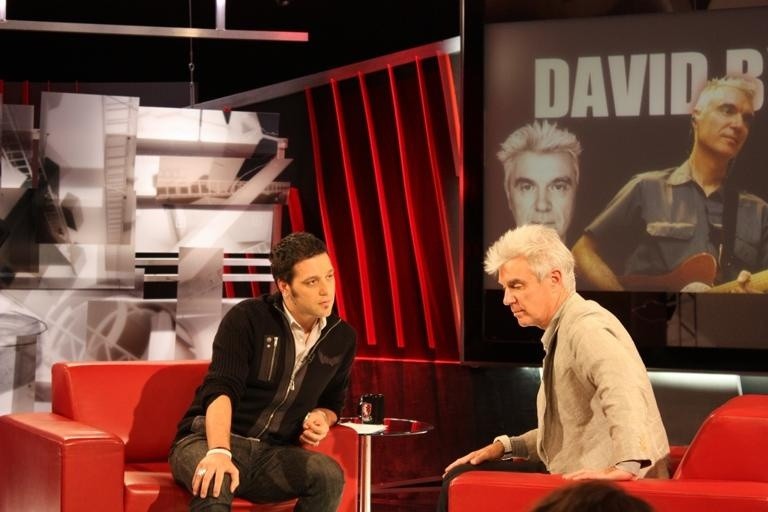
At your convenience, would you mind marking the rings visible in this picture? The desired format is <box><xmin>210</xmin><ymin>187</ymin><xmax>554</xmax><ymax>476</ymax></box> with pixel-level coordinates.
<box><xmin>197</xmin><ymin>467</ymin><xmax>207</xmax><ymax>478</ymax></box>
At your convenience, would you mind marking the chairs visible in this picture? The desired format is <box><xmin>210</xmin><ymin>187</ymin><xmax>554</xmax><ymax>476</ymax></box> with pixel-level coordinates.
<box><xmin>1</xmin><ymin>356</ymin><xmax>371</xmax><ymax>512</ymax></box>
<box><xmin>436</xmin><ymin>394</ymin><xmax>767</xmax><ymax>511</ymax></box>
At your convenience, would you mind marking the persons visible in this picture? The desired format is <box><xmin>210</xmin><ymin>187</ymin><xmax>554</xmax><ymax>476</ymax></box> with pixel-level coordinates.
<box><xmin>437</xmin><ymin>222</ymin><xmax>671</xmax><ymax>512</ymax></box>
<box><xmin>485</xmin><ymin>120</ymin><xmax>593</xmax><ymax>290</ymax></box>
<box><xmin>569</xmin><ymin>75</ymin><xmax>768</xmax><ymax>296</ymax></box>
<box><xmin>164</xmin><ymin>232</ymin><xmax>357</xmax><ymax>512</ymax></box>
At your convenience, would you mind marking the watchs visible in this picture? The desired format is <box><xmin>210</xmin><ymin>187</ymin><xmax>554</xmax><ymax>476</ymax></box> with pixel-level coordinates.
<box><xmin>300</xmin><ymin>407</ymin><xmax>330</xmax><ymax>425</ymax></box>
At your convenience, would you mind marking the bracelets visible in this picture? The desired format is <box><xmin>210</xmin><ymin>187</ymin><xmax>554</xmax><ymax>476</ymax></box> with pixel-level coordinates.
<box><xmin>492</xmin><ymin>434</ymin><xmax>512</xmax><ymax>462</ymax></box>
<box><xmin>205</xmin><ymin>446</ymin><xmax>233</xmax><ymax>461</ymax></box>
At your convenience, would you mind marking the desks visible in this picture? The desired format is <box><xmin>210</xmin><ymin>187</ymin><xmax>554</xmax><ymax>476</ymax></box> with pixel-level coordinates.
<box><xmin>333</xmin><ymin>414</ymin><xmax>434</xmax><ymax>511</ymax></box>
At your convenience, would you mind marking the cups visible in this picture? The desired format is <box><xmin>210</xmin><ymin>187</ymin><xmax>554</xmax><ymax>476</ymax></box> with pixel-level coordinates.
<box><xmin>358</xmin><ymin>394</ymin><xmax>385</xmax><ymax>425</ymax></box>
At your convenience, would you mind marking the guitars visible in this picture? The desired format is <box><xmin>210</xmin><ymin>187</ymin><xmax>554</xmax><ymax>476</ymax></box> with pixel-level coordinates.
<box><xmin>615</xmin><ymin>255</ymin><xmax>768</xmax><ymax>293</ymax></box>
